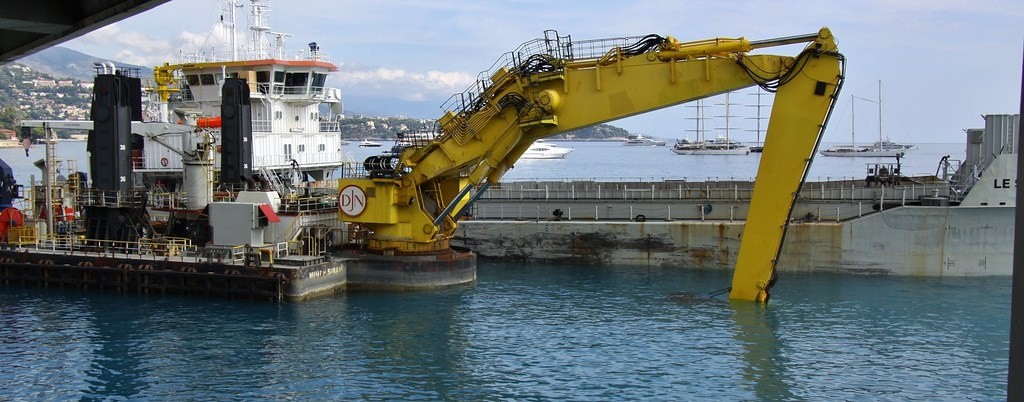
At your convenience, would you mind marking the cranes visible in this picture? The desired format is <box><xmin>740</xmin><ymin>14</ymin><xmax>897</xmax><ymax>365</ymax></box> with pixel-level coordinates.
<box><xmin>330</xmin><ymin>20</ymin><xmax>847</xmax><ymax>306</ymax></box>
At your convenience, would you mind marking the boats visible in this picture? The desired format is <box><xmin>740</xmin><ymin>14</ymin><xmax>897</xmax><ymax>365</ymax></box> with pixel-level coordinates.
<box><xmin>340</xmin><ymin>139</ymin><xmax>353</xmax><ymax>146</ymax></box>
<box><xmin>0</xmin><ymin>0</ymin><xmax>351</xmax><ymax>302</ymax></box>
<box><xmin>620</xmin><ymin>134</ymin><xmax>668</xmax><ymax>146</ymax></box>
<box><xmin>820</xmin><ymin>95</ymin><xmax>918</xmax><ymax>158</ymax></box>
<box><xmin>517</xmin><ymin>138</ymin><xmax>576</xmax><ymax>159</ymax></box>
<box><xmin>394</xmin><ymin>129</ymin><xmax>438</xmax><ymax>148</ymax></box>
<box><xmin>357</xmin><ymin>137</ymin><xmax>383</xmax><ymax>146</ymax></box>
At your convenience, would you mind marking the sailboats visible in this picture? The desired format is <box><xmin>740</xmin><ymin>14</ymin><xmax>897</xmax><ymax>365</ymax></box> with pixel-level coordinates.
<box><xmin>670</xmin><ymin>83</ymin><xmax>773</xmax><ymax>156</ymax></box>
